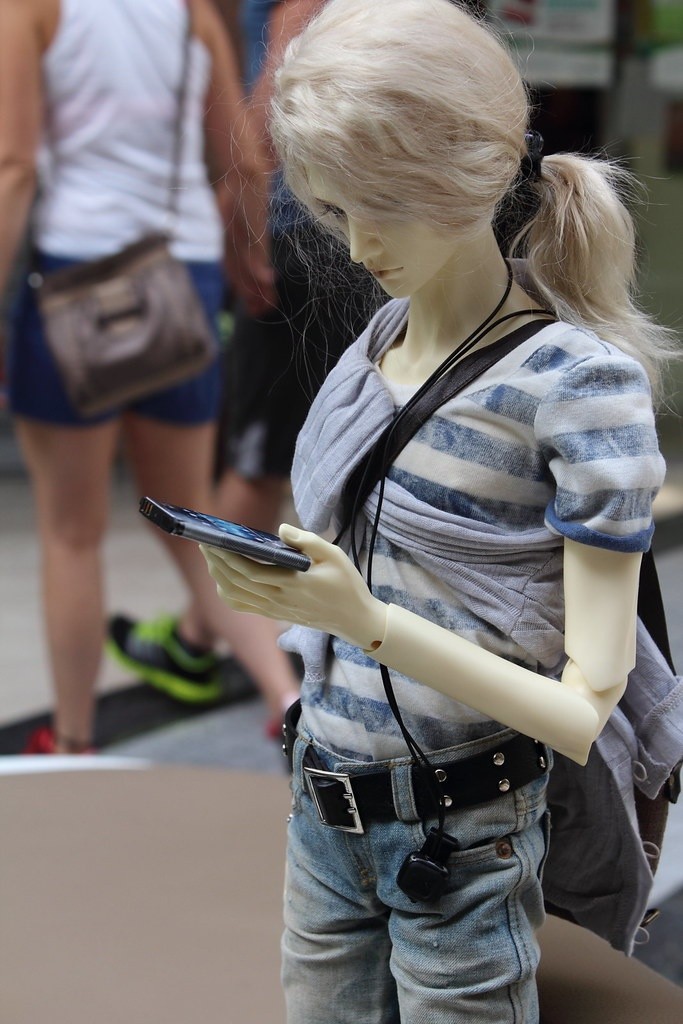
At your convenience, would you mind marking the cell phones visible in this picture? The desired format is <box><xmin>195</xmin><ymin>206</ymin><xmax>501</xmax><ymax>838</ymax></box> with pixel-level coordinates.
<box><xmin>138</xmin><ymin>496</ymin><xmax>312</xmax><ymax>573</ymax></box>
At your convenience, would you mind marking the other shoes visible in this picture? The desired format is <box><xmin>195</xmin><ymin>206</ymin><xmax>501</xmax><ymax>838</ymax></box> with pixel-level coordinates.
<box><xmin>17</xmin><ymin>725</ymin><xmax>97</xmax><ymax>757</ymax></box>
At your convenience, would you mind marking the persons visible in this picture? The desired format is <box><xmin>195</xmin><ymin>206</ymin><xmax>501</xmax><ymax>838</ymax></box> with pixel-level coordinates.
<box><xmin>0</xmin><ymin>1</ymin><xmax>399</xmax><ymax>752</ymax></box>
<box><xmin>195</xmin><ymin>1</ymin><xmax>683</xmax><ymax>1024</ymax></box>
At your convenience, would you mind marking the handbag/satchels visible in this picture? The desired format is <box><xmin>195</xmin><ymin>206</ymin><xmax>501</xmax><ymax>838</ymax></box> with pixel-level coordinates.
<box><xmin>37</xmin><ymin>234</ymin><xmax>221</xmax><ymax>416</ymax></box>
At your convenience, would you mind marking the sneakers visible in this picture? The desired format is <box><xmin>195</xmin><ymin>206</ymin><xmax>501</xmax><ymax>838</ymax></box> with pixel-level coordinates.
<box><xmin>105</xmin><ymin>613</ymin><xmax>225</xmax><ymax>704</ymax></box>
